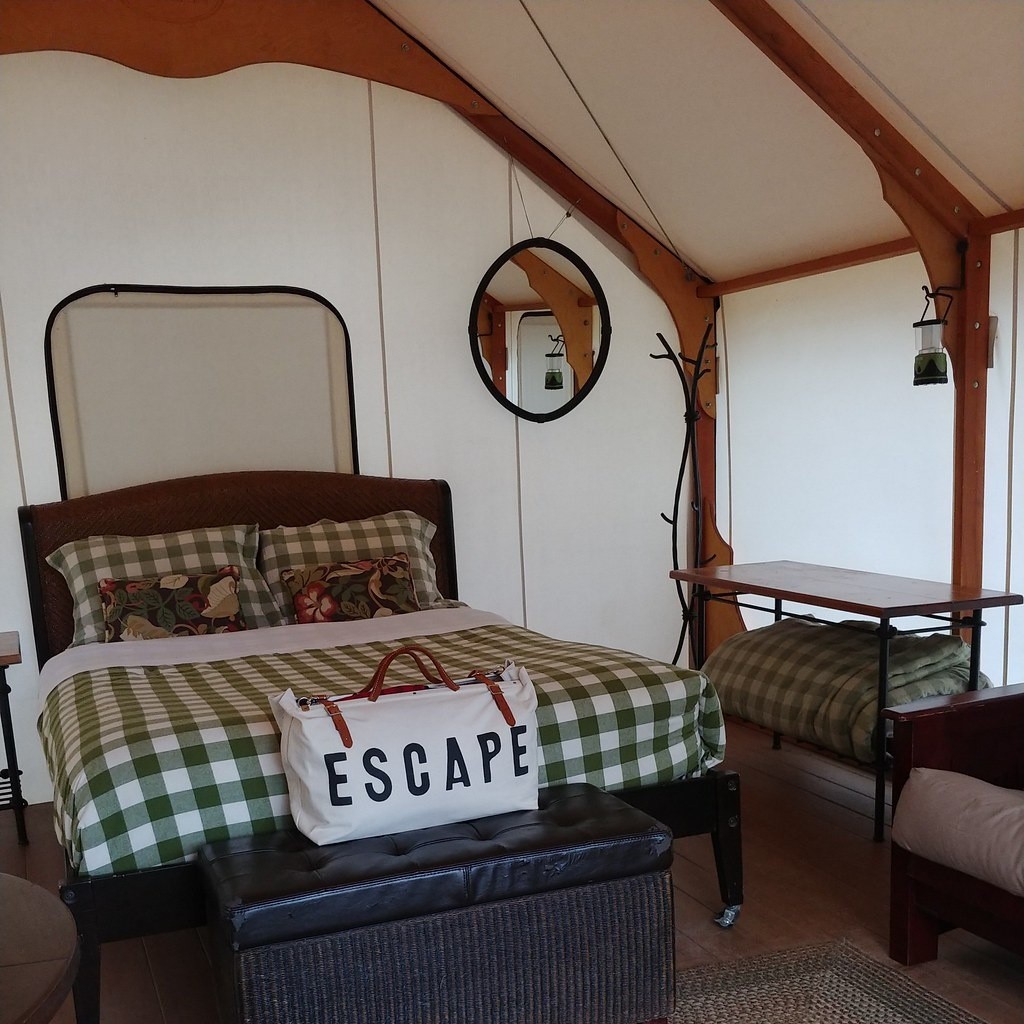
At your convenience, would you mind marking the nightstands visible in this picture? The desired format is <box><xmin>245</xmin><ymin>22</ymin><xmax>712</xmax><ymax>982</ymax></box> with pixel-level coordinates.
<box><xmin>0</xmin><ymin>631</ymin><xmax>32</xmax><ymax>847</ymax></box>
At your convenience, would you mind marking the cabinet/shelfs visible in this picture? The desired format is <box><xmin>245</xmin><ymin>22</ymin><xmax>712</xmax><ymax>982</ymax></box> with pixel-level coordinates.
<box><xmin>670</xmin><ymin>560</ymin><xmax>1024</xmax><ymax>845</ymax></box>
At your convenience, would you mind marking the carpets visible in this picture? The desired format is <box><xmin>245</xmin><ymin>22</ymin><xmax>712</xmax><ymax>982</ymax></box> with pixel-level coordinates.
<box><xmin>673</xmin><ymin>940</ymin><xmax>984</xmax><ymax>1024</ymax></box>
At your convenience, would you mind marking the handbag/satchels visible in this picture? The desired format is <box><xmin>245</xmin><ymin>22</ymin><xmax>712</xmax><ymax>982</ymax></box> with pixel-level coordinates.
<box><xmin>269</xmin><ymin>642</ymin><xmax>541</xmax><ymax>847</ymax></box>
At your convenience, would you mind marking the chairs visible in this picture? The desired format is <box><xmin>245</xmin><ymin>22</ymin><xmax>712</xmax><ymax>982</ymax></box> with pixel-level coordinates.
<box><xmin>884</xmin><ymin>683</ymin><xmax>1024</xmax><ymax>964</ymax></box>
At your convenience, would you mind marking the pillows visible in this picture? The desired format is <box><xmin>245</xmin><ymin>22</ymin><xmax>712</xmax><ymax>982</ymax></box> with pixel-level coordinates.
<box><xmin>279</xmin><ymin>553</ymin><xmax>421</xmax><ymax>627</ymax></box>
<box><xmin>97</xmin><ymin>565</ymin><xmax>248</xmax><ymax>643</ymax></box>
<box><xmin>260</xmin><ymin>510</ymin><xmax>443</xmax><ymax>625</ymax></box>
<box><xmin>46</xmin><ymin>524</ymin><xmax>284</xmax><ymax>649</ymax></box>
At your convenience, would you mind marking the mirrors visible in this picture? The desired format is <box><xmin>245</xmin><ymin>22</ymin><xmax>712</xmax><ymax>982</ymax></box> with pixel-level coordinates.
<box><xmin>468</xmin><ymin>236</ymin><xmax>612</xmax><ymax>422</ymax></box>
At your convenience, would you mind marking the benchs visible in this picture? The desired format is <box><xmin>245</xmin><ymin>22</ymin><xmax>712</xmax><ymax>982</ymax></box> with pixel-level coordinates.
<box><xmin>200</xmin><ymin>782</ymin><xmax>677</xmax><ymax>1024</ymax></box>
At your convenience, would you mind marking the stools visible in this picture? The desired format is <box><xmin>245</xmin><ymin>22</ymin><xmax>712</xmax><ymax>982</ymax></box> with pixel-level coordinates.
<box><xmin>0</xmin><ymin>874</ymin><xmax>103</xmax><ymax>1024</ymax></box>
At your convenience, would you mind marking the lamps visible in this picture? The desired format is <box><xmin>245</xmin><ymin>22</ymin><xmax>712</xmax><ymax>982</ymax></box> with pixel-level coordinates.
<box><xmin>545</xmin><ymin>327</ymin><xmax>567</xmax><ymax>390</ymax></box>
<box><xmin>912</xmin><ymin>281</ymin><xmax>965</xmax><ymax>387</ymax></box>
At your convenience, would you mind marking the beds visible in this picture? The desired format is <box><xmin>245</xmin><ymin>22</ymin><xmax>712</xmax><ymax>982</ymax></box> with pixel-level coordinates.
<box><xmin>16</xmin><ymin>468</ymin><xmax>743</xmax><ymax>946</ymax></box>
